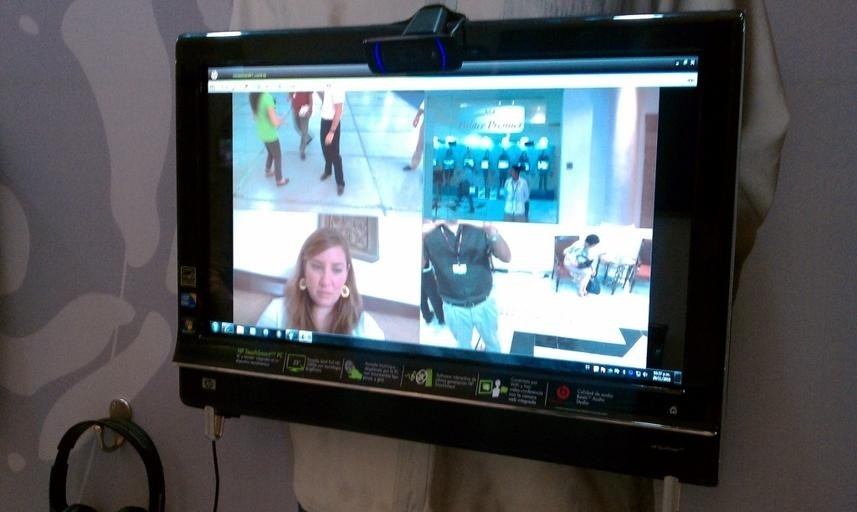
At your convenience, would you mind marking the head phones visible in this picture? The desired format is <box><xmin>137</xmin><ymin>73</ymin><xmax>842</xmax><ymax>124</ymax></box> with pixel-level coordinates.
<box><xmin>49</xmin><ymin>416</ymin><xmax>166</xmax><ymax>512</ymax></box>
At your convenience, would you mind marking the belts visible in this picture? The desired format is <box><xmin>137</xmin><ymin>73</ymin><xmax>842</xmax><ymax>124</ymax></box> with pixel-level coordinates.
<box><xmin>444</xmin><ymin>296</ymin><xmax>488</xmax><ymax>308</ymax></box>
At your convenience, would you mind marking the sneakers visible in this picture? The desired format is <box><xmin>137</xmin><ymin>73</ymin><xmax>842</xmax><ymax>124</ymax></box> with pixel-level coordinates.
<box><xmin>321</xmin><ymin>172</ymin><xmax>344</xmax><ymax>195</ymax></box>
<box><xmin>264</xmin><ymin>168</ymin><xmax>289</xmax><ymax>186</ymax></box>
<box><xmin>299</xmin><ymin>137</ymin><xmax>314</xmax><ymax>161</ymax></box>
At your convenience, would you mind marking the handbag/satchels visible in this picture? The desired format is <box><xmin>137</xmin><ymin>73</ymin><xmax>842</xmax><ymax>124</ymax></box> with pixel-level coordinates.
<box><xmin>586</xmin><ymin>274</ymin><xmax>600</xmax><ymax>294</ymax></box>
<box><xmin>575</xmin><ymin>254</ymin><xmax>593</xmax><ymax>269</ymax></box>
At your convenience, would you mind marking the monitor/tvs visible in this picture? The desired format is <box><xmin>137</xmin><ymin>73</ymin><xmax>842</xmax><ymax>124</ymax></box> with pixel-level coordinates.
<box><xmin>172</xmin><ymin>5</ymin><xmax>747</xmax><ymax>487</ymax></box>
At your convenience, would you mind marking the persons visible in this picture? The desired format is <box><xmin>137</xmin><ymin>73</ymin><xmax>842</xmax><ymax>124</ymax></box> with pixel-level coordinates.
<box><xmin>253</xmin><ymin>231</ymin><xmax>388</xmax><ymax>344</ymax></box>
<box><xmin>415</xmin><ymin>221</ymin><xmax>600</xmax><ymax>353</ymax></box>
<box><xmin>432</xmin><ymin>128</ymin><xmax>561</xmax><ymax>222</ymax></box>
<box><xmin>246</xmin><ymin>89</ymin><xmax>425</xmax><ymax>194</ymax></box>
